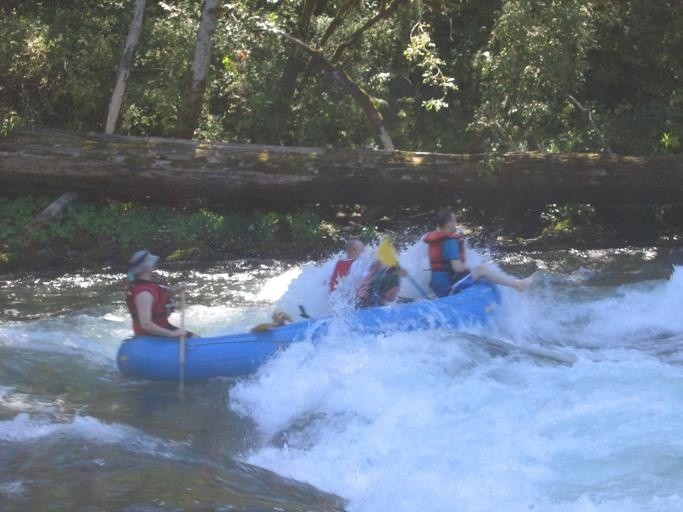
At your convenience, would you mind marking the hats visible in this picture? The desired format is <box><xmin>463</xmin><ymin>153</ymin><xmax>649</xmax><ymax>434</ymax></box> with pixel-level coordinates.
<box><xmin>129</xmin><ymin>249</ymin><xmax>159</xmax><ymax>273</ymax></box>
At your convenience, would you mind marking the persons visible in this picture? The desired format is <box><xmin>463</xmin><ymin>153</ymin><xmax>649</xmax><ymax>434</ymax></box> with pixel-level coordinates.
<box><xmin>419</xmin><ymin>207</ymin><xmax>539</xmax><ymax>299</ymax></box>
<box><xmin>326</xmin><ymin>238</ymin><xmax>366</xmax><ymax>294</ymax></box>
<box><xmin>127</xmin><ymin>247</ymin><xmax>195</xmax><ymax>339</ymax></box>
<box><xmin>351</xmin><ymin>247</ymin><xmax>418</xmax><ymax>313</ymax></box>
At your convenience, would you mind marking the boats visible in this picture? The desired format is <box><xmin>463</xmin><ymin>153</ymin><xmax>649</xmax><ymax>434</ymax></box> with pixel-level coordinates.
<box><xmin>118</xmin><ymin>277</ymin><xmax>499</xmax><ymax>381</ymax></box>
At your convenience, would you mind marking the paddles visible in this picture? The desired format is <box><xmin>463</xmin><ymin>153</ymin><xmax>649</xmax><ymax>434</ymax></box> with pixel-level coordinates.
<box><xmin>378</xmin><ymin>239</ymin><xmax>431</xmax><ymax>302</ymax></box>
<box><xmin>252</xmin><ymin>323</ymin><xmax>277</xmax><ymax>331</ymax></box>
